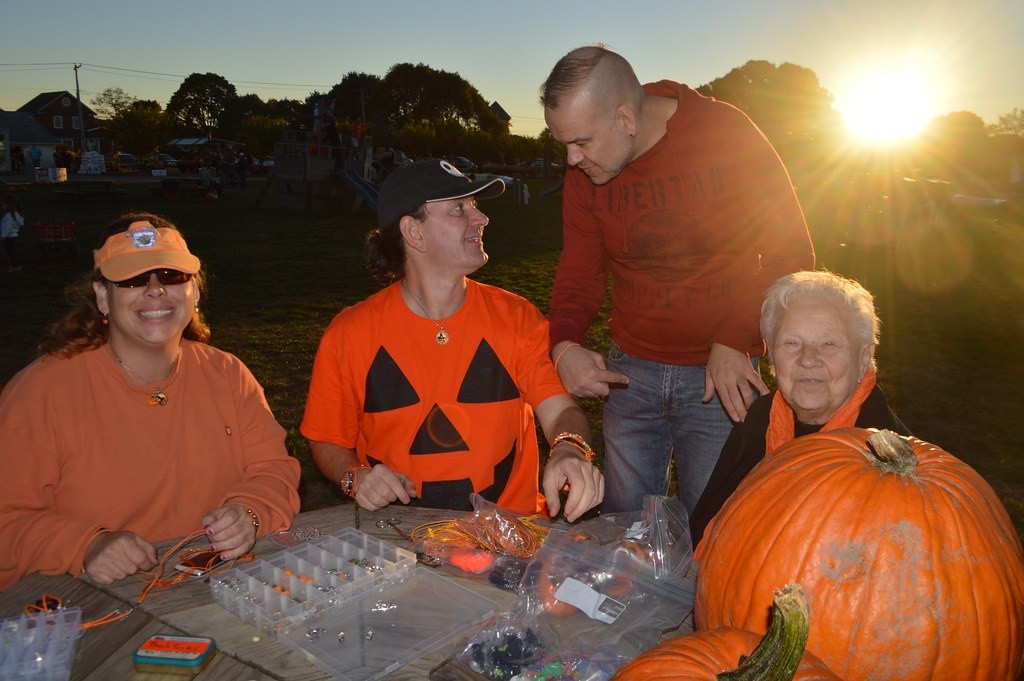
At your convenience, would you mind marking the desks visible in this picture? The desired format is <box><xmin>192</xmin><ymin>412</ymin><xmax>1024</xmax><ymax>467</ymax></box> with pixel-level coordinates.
<box><xmin>0</xmin><ymin>504</ymin><xmax>700</xmax><ymax>681</ymax></box>
<box><xmin>65</xmin><ymin>180</ymin><xmax>112</xmax><ymax>203</ymax></box>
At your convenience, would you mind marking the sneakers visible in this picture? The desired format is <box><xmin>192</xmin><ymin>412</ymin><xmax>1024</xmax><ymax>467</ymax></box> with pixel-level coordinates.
<box><xmin>5</xmin><ymin>264</ymin><xmax>23</xmax><ymax>272</ymax></box>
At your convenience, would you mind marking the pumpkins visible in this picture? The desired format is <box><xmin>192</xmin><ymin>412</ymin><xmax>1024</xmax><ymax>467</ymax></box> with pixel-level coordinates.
<box><xmin>692</xmin><ymin>426</ymin><xmax>1024</xmax><ymax>681</ymax></box>
<box><xmin>607</xmin><ymin>581</ymin><xmax>846</xmax><ymax>681</ymax></box>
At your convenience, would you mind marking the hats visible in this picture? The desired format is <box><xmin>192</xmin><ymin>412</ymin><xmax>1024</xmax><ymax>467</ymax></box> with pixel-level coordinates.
<box><xmin>376</xmin><ymin>158</ymin><xmax>506</xmax><ymax>228</ymax></box>
<box><xmin>92</xmin><ymin>218</ymin><xmax>202</xmax><ymax>282</ymax></box>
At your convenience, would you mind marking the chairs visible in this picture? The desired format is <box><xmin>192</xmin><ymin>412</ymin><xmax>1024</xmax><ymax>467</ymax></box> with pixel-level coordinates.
<box><xmin>34</xmin><ymin>219</ymin><xmax>81</xmax><ymax>262</ymax></box>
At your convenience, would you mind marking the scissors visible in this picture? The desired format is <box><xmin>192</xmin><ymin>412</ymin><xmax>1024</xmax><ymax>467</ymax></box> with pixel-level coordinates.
<box><xmin>376</xmin><ymin>518</ymin><xmax>414</xmax><ymax>542</ymax></box>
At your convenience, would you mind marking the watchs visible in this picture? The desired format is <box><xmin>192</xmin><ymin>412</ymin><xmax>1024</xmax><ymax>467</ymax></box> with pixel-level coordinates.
<box><xmin>341</xmin><ymin>463</ymin><xmax>372</xmax><ymax>498</ymax></box>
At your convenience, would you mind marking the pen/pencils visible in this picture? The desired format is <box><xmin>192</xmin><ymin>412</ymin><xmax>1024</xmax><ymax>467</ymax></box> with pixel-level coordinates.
<box><xmin>355</xmin><ymin>505</ymin><xmax>360</xmax><ymax>530</ymax></box>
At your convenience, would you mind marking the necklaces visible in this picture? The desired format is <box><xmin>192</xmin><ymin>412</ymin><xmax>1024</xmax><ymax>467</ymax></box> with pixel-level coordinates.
<box><xmin>401</xmin><ymin>277</ymin><xmax>467</xmax><ymax>345</ymax></box>
<box><xmin>108</xmin><ymin>339</ymin><xmax>180</xmax><ymax>406</ymax></box>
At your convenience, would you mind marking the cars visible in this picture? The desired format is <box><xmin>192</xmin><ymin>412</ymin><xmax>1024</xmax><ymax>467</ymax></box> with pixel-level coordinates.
<box><xmin>111</xmin><ymin>149</ymin><xmax>142</xmax><ymax>173</ymax></box>
<box><xmin>447</xmin><ymin>153</ymin><xmax>480</xmax><ymax>174</ymax></box>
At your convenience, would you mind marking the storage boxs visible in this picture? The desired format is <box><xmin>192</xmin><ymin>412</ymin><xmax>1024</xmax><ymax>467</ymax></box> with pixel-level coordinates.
<box><xmin>0</xmin><ymin>603</ymin><xmax>82</xmax><ymax>681</ymax></box>
<box><xmin>208</xmin><ymin>525</ymin><xmax>502</xmax><ymax>681</ymax></box>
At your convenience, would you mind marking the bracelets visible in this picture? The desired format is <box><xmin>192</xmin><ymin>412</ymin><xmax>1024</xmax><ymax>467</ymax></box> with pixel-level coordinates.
<box><xmin>547</xmin><ymin>432</ymin><xmax>605</xmax><ymax>491</ymax></box>
<box><xmin>243</xmin><ymin>506</ymin><xmax>257</xmax><ymax>530</ymax></box>
<box><xmin>555</xmin><ymin>344</ymin><xmax>582</xmax><ymax>373</ymax></box>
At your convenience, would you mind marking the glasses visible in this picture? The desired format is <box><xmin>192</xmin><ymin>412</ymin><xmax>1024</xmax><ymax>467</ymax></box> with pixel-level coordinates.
<box><xmin>107</xmin><ymin>269</ymin><xmax>191</xmax><ymax>289</ymax></box>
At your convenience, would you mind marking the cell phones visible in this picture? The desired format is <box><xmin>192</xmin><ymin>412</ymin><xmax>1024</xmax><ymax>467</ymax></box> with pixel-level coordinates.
<box><xmin>174</xmin><ymin>551</ymin><xmax>228</xmax><ymax>577</ymax></box>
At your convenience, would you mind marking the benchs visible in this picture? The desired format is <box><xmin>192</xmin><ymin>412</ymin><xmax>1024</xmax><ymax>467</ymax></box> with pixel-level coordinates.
<box><xmin>56</xmin><ymin>190</ymin><xmax>132</xmax><ymax>203</ymax></box>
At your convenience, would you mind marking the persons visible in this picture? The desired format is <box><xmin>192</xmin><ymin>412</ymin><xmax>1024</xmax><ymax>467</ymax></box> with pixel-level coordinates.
<box><xmin>690</xmin><ymin>270</ymin><xmax>916</xmax><ymax>554</ymax></box>
<box><xmin>306</xmin><ymin>135</ymin><xmax>318</xmax><ymax>176</ymax></box>
<box><xmin>379</xmin><ymin>143</ymin><xmax>403</xmax><ymax>177</ymax></box>
<box><xmin>28</xmin><ymin>143</ymin><xmax>42</xmax><ymax>168</ymax></box>
<box><xmin>542</xmin><ymin>46</ymin><xmax>816</xmax><ymax>525</ymax></box>
<box><xmin>303</xmin><ymin>159</ymin><xmax>607</xmax><ymax>519</ymax></box>
<box><xmin>1</xmin><ymin>214</ymin><xmax>300</xmax><ymax>587</ymax></box>
